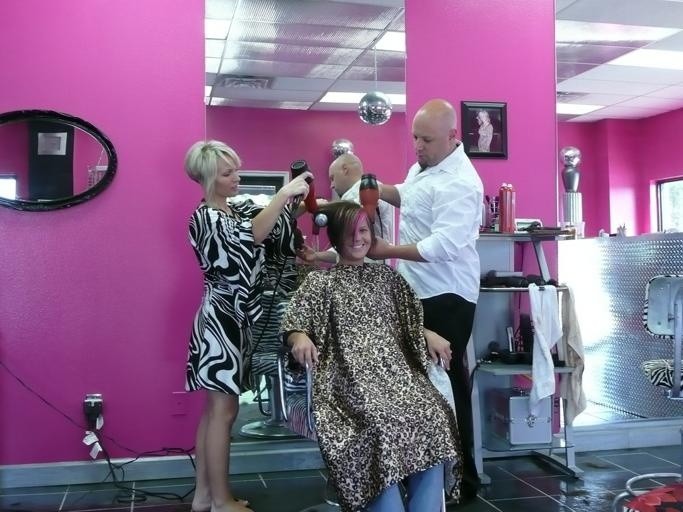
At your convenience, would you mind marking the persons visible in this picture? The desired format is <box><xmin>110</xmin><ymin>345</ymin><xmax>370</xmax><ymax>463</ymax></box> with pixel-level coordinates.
<box><xmin>475</xmin><ymin>111</ymin><xmax>494</xmax><ymax>152</ymax></box>
<box><xmin>365</xmin><ymin>98</ymin><xmax>485</xmax><ymax>507</ymax></box>
<box><xmin>183</xmin><ymin>139</ymin><xmax>316</xmax><ymax>512</ymax></box>
<box><xmin>294</xmin><ymin>153</ymin><xmax>395</xmax><ymax>269</ymax></box>
<box><xmin>279</xmin><ymin>201</ymin><xmax>460</xmax><ymax>512</ymax></box>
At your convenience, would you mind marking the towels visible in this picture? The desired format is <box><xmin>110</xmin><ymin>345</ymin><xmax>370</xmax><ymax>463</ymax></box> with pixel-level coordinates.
<box><xmin>523</xmin><ymin>282</ymin><xmax>587</xmax><ymax>427</ymax></box>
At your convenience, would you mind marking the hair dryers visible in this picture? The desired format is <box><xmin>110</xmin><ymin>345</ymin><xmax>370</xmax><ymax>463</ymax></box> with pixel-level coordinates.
<box><xmin>487</xmin><ymin>340</ymin><xmax>501</xmax><ymax>362</ymax></box>
<box><xmin>290</xmin><ymin>156</ymin><xmax>318</xmax><ymax>213</ymax></box>
<box><xmin>359</xmin><ymin>172</ymin><xmax>380</xmax><ymax>226</ymax></box>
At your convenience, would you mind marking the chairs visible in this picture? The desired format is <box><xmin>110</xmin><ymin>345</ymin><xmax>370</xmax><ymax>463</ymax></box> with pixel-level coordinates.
<box><xmin>238</xmin><ymin>288</ymin><xmax>304</xmax><ymax>441</ymax></box>
<box><xmin>638</xmin><ymin>274</ymin><xmax>683</xmax><ymax>402</ymax></box>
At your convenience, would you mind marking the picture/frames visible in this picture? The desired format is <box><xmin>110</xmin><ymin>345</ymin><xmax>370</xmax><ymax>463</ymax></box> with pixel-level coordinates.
<box><xmin>458</xmin><ymin>97</ymin><xmax>509</xmax><ymax>161</ymax></box>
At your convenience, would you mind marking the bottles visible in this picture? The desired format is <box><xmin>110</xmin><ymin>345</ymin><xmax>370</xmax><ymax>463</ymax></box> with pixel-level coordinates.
<box><xmin>499</xmin><ymin>181</ymin><xmax>518</xmax><ymax>234</ymax></box>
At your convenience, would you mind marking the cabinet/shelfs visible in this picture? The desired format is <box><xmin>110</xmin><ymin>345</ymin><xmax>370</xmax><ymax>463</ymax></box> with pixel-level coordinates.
<box><xmin>454</xmin><ymin>226</ymin><xmax>586</xmax><ymax>490</ymax></box>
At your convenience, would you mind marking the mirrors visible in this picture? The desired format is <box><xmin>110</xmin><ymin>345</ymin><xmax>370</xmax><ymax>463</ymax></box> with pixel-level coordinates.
<box><xmin>554</xmin><ymin>1</ymin><xmax>683</xmax><ymax>434</ymax></box>
<box><xmin>203</xmin><ymin>0</ymin><xmax>408</xmax><ymax>453</ymax></box>
<box><xmin>0</xmin><ymin>108</ymin><xmax>118</xmax><ymax>212</ymax></box>
